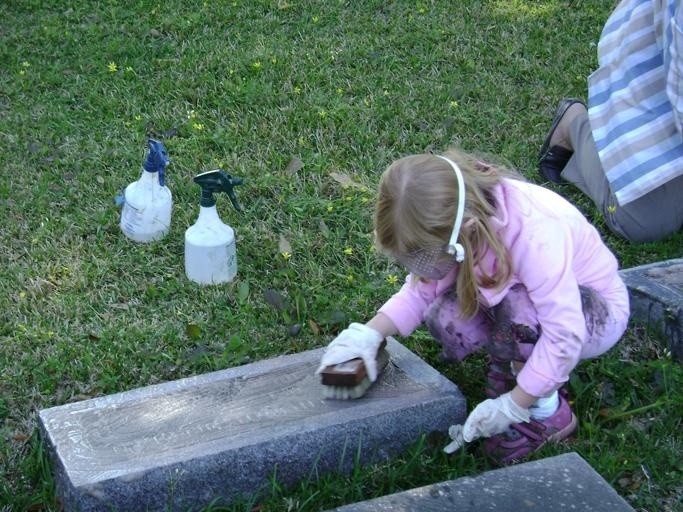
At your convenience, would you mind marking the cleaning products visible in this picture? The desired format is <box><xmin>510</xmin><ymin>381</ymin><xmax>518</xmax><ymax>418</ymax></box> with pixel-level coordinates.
<box><xmin>119</xmin><ymin>138</ymin><xmax>175</xmax><ymax>244</ymax></box>
<box><xmin>185</xmin><ymin>169</ymin><xmax>244</xmax><ymax>286</ymax></box>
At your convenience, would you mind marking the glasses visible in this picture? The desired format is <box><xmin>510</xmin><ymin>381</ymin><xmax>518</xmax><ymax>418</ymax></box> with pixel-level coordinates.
<box><xmin>386</xmin><ymin>244</ymin><xmax>459</xmax><ymax>281</ymax></box>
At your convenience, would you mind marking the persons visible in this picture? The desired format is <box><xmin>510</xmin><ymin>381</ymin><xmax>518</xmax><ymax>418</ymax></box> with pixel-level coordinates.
<box><xmin>314</xmin><ymin>141</ymin><xmax>633</xmax><ymax>470</ymax></box>
<box><xmin>534</xmin><ymin>0</ymin><xmax>683</xmax><ymax>247</ymax></box>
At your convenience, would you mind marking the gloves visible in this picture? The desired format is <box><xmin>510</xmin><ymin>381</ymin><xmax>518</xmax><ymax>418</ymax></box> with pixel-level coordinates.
<box><xmin>443</xmin><ymin>392</ymin><xmax>530</xmax><ymax>455</ymax></box>
<box><xmin>315</xmin><ymin>322</ymin><xmax>384</xmax><ymax>382</ymax></box>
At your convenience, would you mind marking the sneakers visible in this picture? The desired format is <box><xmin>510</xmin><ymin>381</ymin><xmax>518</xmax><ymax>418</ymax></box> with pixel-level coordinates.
<box><xmin>484</xmin><ymin>354</ymin><xmax>517</xmax><ymax>397</ymax></box>
<box><xmin>481</xmin><ymin>394</ymin><xmax>577</xmax><ymax>467</ymax></box>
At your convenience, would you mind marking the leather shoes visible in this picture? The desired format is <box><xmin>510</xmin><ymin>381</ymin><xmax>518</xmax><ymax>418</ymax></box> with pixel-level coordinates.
<box><xmin>538</xmin><ymin>98</ymin><xmax>588</xmax><ymax>185</ymax></box>
